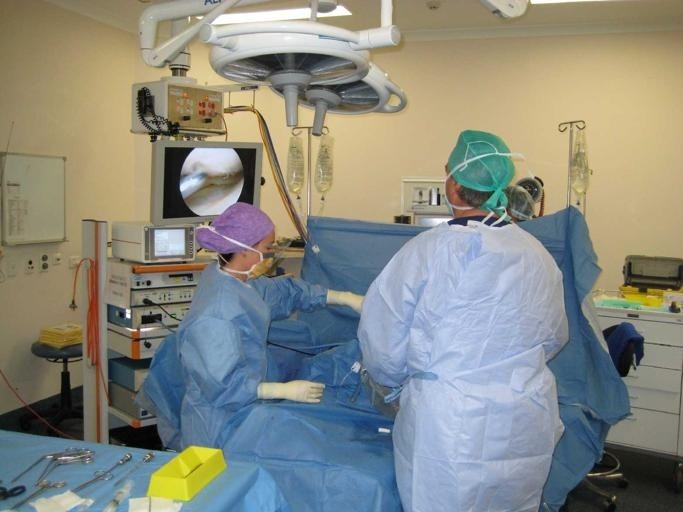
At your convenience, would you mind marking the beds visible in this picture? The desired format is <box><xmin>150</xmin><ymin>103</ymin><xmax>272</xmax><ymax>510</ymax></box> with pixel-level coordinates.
<box><xmin>216</xmin><ymin>201</ymin><xmax>629</xmax><ymax>512</ymax></box>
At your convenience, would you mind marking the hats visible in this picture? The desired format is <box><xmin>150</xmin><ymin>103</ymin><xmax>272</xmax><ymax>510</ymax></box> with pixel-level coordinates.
<box><xmin>448</xmin><ymin>130</ymin><xmax>515</xmax><ymax>223</ymax></box>
<box><xmin>194</xmin><ymin>202</ymin><xmax>275</xmax><ymax>254</ymax></box>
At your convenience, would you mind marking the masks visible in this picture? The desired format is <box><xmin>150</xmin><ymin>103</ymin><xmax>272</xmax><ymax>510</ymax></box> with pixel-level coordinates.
<box><xmin>244</xmin><ymin>247</ymin><xmax>275</xmax><ymax>280</ymax></box>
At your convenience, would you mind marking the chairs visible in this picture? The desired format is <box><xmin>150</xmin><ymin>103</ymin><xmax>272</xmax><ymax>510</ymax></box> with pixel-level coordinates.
<box><xmin>575</xmin><ymin>317</ymin><xmax>645</xmax><ymax>511</ymax></box>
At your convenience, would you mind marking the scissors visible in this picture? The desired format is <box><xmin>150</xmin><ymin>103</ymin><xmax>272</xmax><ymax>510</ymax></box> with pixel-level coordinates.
<box><xmin>71</xmin><ymin>471</ymin><xmax>113</xmax><ymax>492</ymax></box>
<box><xmin>0</xmin><ymin>486</ymin><xmax>25</xmax><ymax>502</ymax></box>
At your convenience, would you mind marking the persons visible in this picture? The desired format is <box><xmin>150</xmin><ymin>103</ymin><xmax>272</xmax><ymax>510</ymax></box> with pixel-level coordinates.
<box><xmin>156</xmin><ymin>203</ymin><xmax>365</xmax><ymax>452</ymax></box>
<box><xmin>502</xmin><ymin>185</ymin><xmax>535</xmax><ymax>222</ymax></box>
<box><xmin>358</xmin><ymin>128</ymin><xmax>570</xmax><ymax>510</ymax></box>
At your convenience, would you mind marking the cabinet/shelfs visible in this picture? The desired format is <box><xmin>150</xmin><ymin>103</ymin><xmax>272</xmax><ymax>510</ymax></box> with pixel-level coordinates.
<box><xmin>582</xmin><ymin>286</ymin><xmax>682</xmax><ymax>498</ymax></box>
<box><xmin>71</xmin><ymin>247</ymin><xmax>216</xmax><ymax>441</ymax></box>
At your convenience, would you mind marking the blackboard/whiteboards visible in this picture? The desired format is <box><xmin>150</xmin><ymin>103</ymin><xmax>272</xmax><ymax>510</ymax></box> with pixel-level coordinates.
<box><xmin>0</xmin><ymin>152</ymin><xmax>67</xmax><ymax>247</ymax></box>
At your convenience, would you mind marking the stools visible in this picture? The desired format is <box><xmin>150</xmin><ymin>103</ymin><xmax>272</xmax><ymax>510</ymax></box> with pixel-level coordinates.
<box><xmin>15</xmin><ymin>336</ymin><xmax>90</xmax><ymax>441</ymax></box>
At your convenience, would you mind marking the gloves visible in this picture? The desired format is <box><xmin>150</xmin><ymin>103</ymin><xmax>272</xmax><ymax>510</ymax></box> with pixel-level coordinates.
<box><xmin>326</xmin><ymin>289</ymin><xmax>364</xmax><ymax>315</ymax></box>
<box><xmin>257</xmin><ymin>379</ymin><xmax>326</xmax><ymax>403</ymax></box>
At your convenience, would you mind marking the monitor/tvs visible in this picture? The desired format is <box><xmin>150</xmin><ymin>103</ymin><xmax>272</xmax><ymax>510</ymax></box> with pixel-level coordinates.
<box><xmin>150</xmin><ymin>140</ymin><xmax>263</xmax><ymax>225</ymax></box>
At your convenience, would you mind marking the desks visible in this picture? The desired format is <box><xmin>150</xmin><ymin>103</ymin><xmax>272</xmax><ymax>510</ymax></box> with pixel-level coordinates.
<box><xmin>0</xmin><ymin>429</ymin><xmax>294</xmax><ymax>511</ymax></box>
